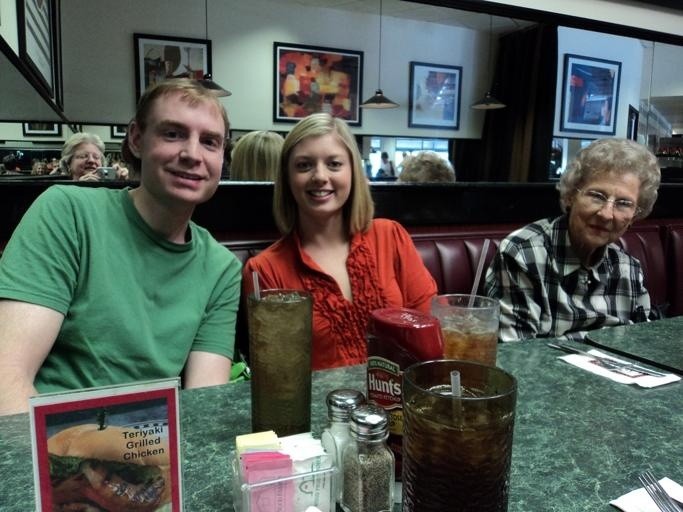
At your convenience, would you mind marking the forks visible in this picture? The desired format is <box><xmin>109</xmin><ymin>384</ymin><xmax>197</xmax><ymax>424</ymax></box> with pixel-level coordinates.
<box><xmin>639</xmin><ymin>471</ymin><xmax>683</xmax><ymax>512</ymax></box>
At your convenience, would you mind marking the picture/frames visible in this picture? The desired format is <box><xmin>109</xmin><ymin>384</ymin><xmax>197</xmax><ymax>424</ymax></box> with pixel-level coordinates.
<box><xmin>560</xmin><ymin>53</ymin><xmax>622</xmax><ymax>136</ymax></box>
<box><xmin>272</xmin><ymin>42</ymin><xmax>364</xmax><ymax>126</ymax></box>
<box><xmin>409</xmin><ymin>61</ymin><xmax>462</xmax><ymax>130</ymax></box>
<box><xmin>132</xmin><ymin>32</ymin><xmax>212</xmax><ymax>110</ymax></box>
<box><xmin>110</xmin><ymin>125</ymin><xmax>128</xmax><ymax>139</ymax></box>
<box><xmin>22</xmin><ymin>121</ymin><xmax>62</xmax><ymax>136</ymax></box>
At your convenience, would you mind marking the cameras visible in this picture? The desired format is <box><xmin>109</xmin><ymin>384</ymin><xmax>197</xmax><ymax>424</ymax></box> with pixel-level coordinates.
<box><xmin>97</xmin><ymin>166</ymin><xmax>118</xmax><ymax>180</ymax></box>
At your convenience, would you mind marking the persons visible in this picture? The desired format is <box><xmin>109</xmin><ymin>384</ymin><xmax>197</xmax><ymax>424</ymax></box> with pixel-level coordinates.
<box><xmin>60</xmin><ymin>132</ymin><xmax>129</xmax><ymax>182</ymax></box>
<box><xmin>0</xmin><ymin>78</ymin><xmax>243</xmax><ymax>416</ymax></box>
<box><xmin>396</xmin><ymin>151</ymin><xmax>407</xmax><ymax>170</ymax></box>
<box><xmin>380</xmin><ymin>152</ymin><xmax>399</xmax><ymax>177</ymax></box>
<box><xmin>484</xmin><ymin>137</ymin><xmax>662</xmax><ymax>342</ymax></box>
<box><xmin>376</xmin><ymin>168</ymin><xmax>388</xmax><ymax>178</ymax></box>
<box><xmin>397</xmin><ymin>152</ymin><xmax>455</xmax><ymax>182</ymax></box>
<box><xmin>242</xmin><ymin>113</ymin><xmax>449</xmax><ymax>371</ymax></box>
<box><xmin>1</xmin><ymin>153</ymin><xmax>66</xmax><ymax>176</ymax></box>
<box><xmin>223</xmin><ymin>130</ymin><xmax>285</xmax><ymax>181</ymax></box>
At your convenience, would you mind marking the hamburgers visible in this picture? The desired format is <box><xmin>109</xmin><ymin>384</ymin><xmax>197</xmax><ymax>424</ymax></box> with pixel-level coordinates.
<box><xmin>45</xmin><ymin>419</ymin><xmax>171</xmax><ymax>510</ymax></box>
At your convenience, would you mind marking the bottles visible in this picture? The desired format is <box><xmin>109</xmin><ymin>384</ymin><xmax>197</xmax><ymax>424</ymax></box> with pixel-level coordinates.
<box><xmin>342</xmin><ymin>404</ymin><xmax>395</xmax><ymax>512</ymax></box>
<box><xmin>321</xmin><ymin>389</ymin><xmax>366</xmax><ymax>505</ymax></box>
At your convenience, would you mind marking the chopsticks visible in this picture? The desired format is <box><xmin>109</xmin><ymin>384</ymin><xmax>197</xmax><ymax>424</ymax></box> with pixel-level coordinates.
<box><xmin>550</xmin><ymin>343</ymin><xmax>666</xmax><ymax>377</ymax></box>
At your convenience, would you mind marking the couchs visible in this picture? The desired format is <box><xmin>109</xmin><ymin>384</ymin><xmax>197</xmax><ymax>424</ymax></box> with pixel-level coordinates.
<box><xmin>216</xmin><ymin>219</ymin><xmax>682</xmax><ymax>359</ymax></box>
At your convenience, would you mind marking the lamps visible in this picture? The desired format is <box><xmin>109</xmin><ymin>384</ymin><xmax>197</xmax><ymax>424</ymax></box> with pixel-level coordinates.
<box><xmin>360</xmin><ymin>1</ymin><xmax>399</xmax><ymax>110</ymax></box>
<box><xmin>469</xmin><ymin>14</ymin><xmax>507</xmax><ymax>110</ymax></box>
<box><xmin>195</xmin><ymin>1</ymin><xmax>231</xmax><ymax>97</ymax></box>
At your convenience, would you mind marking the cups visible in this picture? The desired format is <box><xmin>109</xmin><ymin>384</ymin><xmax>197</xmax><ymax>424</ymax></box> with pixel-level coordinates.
<box><xmin>430</xmin><ymin>293</ymin><xmax>500</xmax><ymax>367</ymax></box>
<box><xmin>401</xmin><ymin>360</ymin><xmax>517</xmax><ymax>512</ymax></box>
<box><xmin>247</xmin><ymin>288</ymin><xmax>314</xmax><ymax>437</ymax></box>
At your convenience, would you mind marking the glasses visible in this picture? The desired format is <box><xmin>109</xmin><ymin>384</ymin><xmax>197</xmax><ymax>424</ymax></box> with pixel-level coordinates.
<box><xmin>575</xmin><ymin>189</ymin><xmax>643</xmax><ymax>217</ymax></box>
